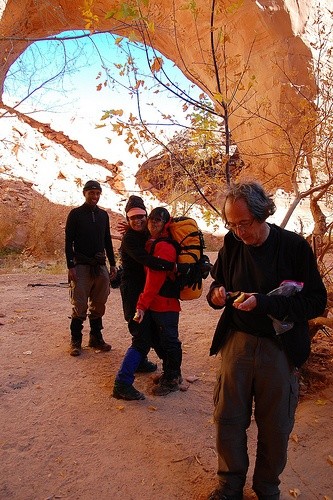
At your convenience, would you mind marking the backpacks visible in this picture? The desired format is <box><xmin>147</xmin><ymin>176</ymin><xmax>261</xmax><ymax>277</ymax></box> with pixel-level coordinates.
<box><xmin>150</xmin><ymin>216</ymin><xmax>204</xmax><ymax>300</ymax></box>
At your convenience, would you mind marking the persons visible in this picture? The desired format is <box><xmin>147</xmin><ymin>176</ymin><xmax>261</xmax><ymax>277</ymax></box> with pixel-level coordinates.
<box><xmin>64</xmin><ymin>180</ymin><xmax>117</xmax><ymax>357</ymax></box>
<box><xmin>110</xmin><ymin>195</ymin><xmax>194</xmax><ymax>401</ymax></box>
<box><xmin>205</xmin><ymin>181</ymin><xmax>328</xmax><ymax>500</ymax></box>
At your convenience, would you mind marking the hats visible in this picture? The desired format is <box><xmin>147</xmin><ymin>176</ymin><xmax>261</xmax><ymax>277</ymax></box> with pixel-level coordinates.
<box><xmin>83</xmin><ymin>180</ymin><xmax>102</xmax><ymax>193</ymax></box>
<box><xmin>126</xmin><ymin>208</ymin><xmax>146</xmax><ymax>218</ymax></box>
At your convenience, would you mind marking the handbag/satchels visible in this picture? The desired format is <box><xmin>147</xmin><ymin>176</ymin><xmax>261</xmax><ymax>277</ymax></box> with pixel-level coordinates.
<box><xmin>109</xmin><ymin>266</ymin><xmax>123</xmax><ymax>289</ymax></box>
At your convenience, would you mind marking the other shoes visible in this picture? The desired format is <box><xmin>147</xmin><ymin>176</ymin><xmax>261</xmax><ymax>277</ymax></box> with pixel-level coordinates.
<box><xmin>112</xmin><ymin>381</ymin><xmax>146</xmax><ymax>401</ymax></box>
<box><xmin>135</xmin><ymin>357</ymin><xmax>157</xmax><ymax>374</ymax></box>
<box><xmin>152</xmin><ymin>381</ymin><xmax>180</xmax><ymax>396</ymax></box>
<box><xmin>207</xmin><ymin>489</ymin><xmax>244</xmax><ymax>500</ymax></box>
<box><xmin>153</xmin><ymin>373</ymin><xmax>183</xmax><ymax>386</ymax></box>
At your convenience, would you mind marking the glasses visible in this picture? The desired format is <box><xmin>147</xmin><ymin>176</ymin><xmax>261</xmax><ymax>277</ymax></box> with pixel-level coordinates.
<box><xmin>128</xmin><ymin>215</ymin><xmax>145</xmax><ymax>221</ymax></box>
<box><xmin>148</xmin><ymin>215</ymin><xmax>166</xmax><ymax>223</ymax></box>
<box><xmin>224</xmin><ymin>214</ymin><xmax>256</xmax><ymax>231</ymax></box>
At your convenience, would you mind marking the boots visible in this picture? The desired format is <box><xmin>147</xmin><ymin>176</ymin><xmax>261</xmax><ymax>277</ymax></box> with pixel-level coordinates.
<box><xmin>88</xmin><ymin>331</ymin><xmax>112</xmax><ymax>351</ymax></box>
<box><xmin>69</xmin><ymin>334</ymin><xmax>82</xmax><ymax>356</ymax></box>
